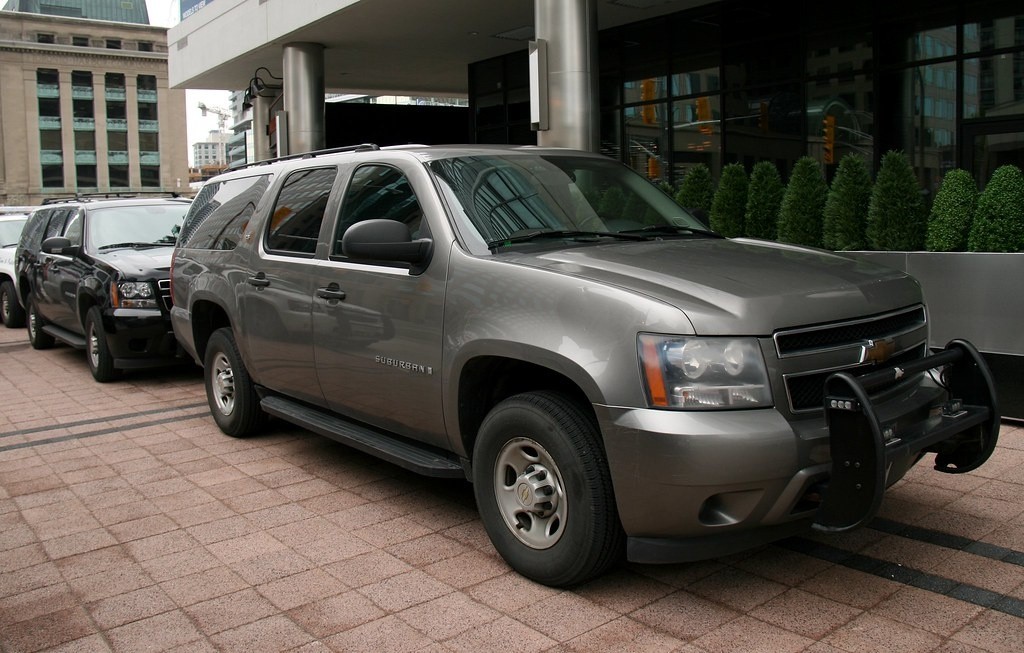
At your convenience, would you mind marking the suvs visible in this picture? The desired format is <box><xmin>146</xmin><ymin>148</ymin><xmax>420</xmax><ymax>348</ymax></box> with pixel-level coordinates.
<box><xmin>169</xmin><ymin>142</ymin><xmax>1001</xmax><ymax>591</ymax></box>
<box><xmin>0</xmin><ymin>205</ymin><xmax>44</xmax><ymax>328</ymax></box>
<box><xmin>14</xmin><ymin>188</ymin><xmax>197</xmax><ymax>383</ymax></box>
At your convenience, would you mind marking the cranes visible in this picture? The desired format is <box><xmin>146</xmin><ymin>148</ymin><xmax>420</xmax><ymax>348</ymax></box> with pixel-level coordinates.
<box><xmin>199</xmin><ymin>102</ymin><xmax>233</xmax><ymax>167</ymax></box>
